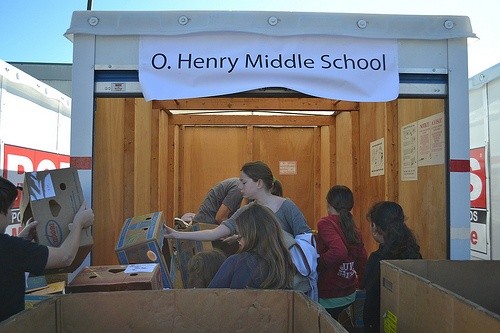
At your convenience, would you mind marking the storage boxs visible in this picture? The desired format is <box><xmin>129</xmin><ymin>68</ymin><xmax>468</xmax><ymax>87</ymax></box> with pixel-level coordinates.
<box><xmin>351</xmin><ymin>288</ymin><xmax>368</xmax><ymax>330</ymax></box>
<box><xmin>19</xmin><ymin>167</ymin><xmax>94</xmax><ymax>274</ymax></box>
<box><xmin>0</xmin><ymin>289</ymin><xmax>349</xmax><ymax>333</ymax></box>
<box><xmin>114</xmin><ymin>212</ymin><xmax>180</xmax><ymax>290</ymax></box>
<box><xmin>168</xmin><ymin>223</ymin><xmax>223</xmax><ymax>277</ymax></box>
<box><xmin>380</xmin><ymin>260</ymin><xmax>500</xmax><ymax>333</ymax></box>
<box><xmin>24</xmin><ymin>274</ymin><xmax>68</xmax><ymax>312</ymax></box>
<box><xmin>65</xmin><ymin>263</ymin><xmax>164</xmax><ymax>292</ymax></box>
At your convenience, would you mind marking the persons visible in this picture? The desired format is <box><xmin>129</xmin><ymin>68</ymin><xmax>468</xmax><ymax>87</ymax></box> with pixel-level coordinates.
<box><xmin>363</xmin><ymin>201</ymin><xmax>424</xmax><ymax>333</ymax></box>
<box><xmin>194</xmin><ymin>177</ymin><xmax>249</xmax><ymax>225</ymax></box>
<box><xmin>206</xmin><ymin>204</ymin><xmax>296</xmax><ymax>290</ymax></box>
<box><xmin>315</xmin><ymin>184</ymin><xmax>368</xmax><ymax>322</ymax></box>
<box><xmin>163</xmin><ymin>161</ymin><xmax>318</xmax><ymax>304</ymax></box>
<box><xmin>0</xmin><ymin>176</ymin><xmax>94</xmax><ymax>321</ymax></box>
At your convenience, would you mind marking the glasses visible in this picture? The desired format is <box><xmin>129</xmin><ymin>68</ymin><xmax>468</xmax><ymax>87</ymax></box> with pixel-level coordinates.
<box><xmin>236</xmin><ymin>237</ymin><xmax>242</xmax><ymax>242</ymax></box>
